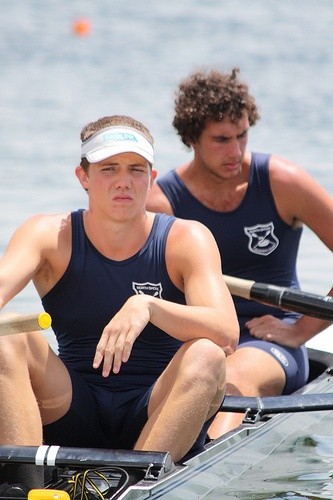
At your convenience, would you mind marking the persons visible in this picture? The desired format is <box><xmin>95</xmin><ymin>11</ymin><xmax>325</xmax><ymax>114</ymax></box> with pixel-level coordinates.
<box><xmin>145</xmin><ymin>66</ymin><xmax>333</xmax><ymax>445</ymax></box>
<box><xmin>0</xmin><ymin>115</ymin><xmax>239</xmax><ymax>499</ymax></box>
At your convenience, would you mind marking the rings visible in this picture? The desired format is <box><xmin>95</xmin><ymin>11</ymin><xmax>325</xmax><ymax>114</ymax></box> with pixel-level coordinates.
<box><xmin>266</xmin><ymin>333</ymin><xmax>271</xmax><ymax>340</ymax></box>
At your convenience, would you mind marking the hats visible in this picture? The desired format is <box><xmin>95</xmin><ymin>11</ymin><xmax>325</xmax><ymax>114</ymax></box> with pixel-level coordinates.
<box><xmin>80</xmin><ymin>126</ymin><xmax>156</xmax><ymax>168</ymax></box>
